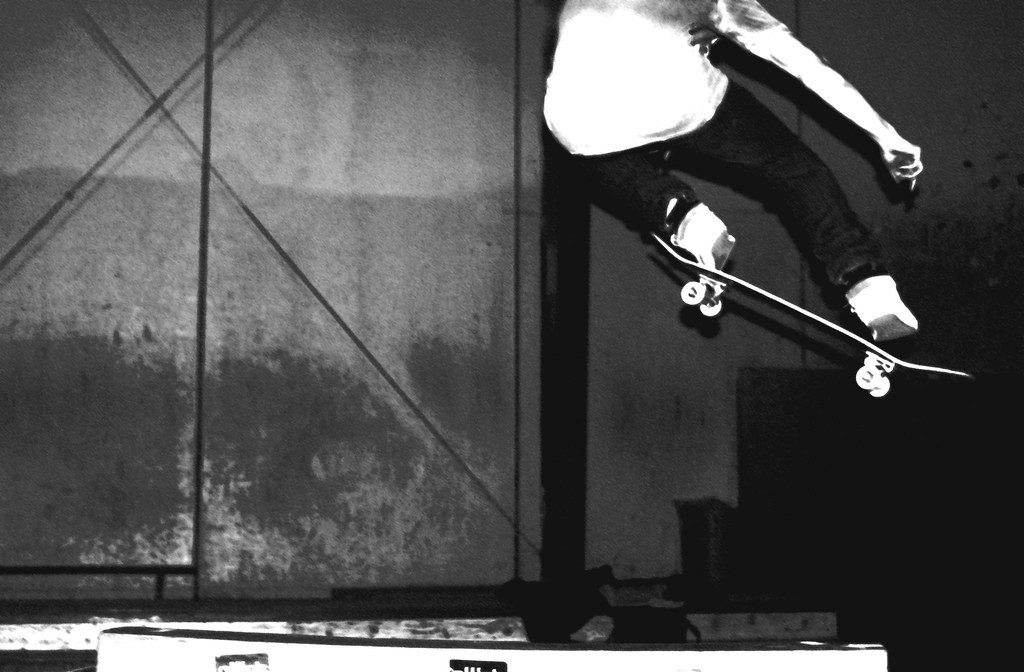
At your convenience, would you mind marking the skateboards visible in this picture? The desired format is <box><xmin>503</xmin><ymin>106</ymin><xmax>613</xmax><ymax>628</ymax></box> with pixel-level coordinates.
<box><xmin>648</xmin><ymin>231</ymin><xmax>976</xmax><ymax>398</ymax></box>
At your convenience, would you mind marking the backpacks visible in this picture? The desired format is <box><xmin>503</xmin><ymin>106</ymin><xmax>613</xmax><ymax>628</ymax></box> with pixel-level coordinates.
<box><xmin>521</xmin><ymin>565</ymin><xmax>701</xmax><ymax>643</ymax></box>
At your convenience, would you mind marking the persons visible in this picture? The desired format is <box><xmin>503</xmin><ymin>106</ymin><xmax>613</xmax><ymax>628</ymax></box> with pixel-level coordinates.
<box><xmin>541</xmin><ymin>1</ymin><xmax>925</xmax><ymax>343</ymax></box>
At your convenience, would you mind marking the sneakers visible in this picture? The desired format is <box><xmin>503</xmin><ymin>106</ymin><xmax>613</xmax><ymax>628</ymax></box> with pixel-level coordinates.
<box><xmin>670</xmin><ymin>202</ymin><xmax>736</xmax><ymax>270</ymax></box>
<box><xmin>846</xmin><ymin>275</ymin><xmax>918</xmax><ymax>341</ymax></box>
